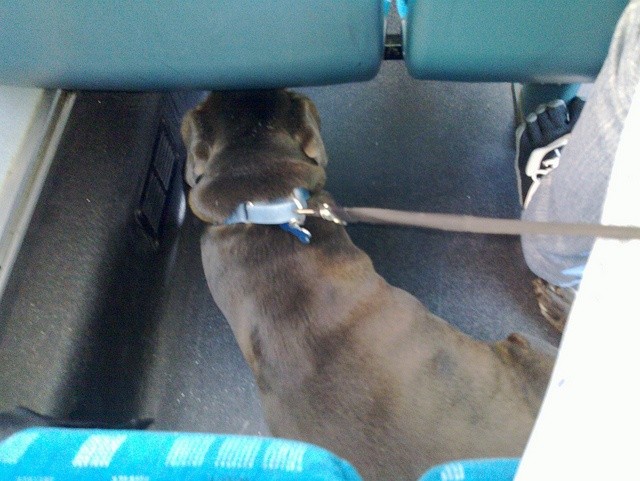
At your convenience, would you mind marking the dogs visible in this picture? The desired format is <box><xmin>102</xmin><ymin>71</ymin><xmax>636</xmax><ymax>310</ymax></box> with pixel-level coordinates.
<box><xmin>181</xmin><ymin>87</ymin><xmax>558</xmax><ymax>481</ymax></box>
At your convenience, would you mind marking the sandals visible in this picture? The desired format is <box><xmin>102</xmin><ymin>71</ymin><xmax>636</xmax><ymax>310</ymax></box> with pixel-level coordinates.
<box><xmin>512</xmin><ymin>94</ymin><xmax>587</xmax><ymax>332</ymax></box>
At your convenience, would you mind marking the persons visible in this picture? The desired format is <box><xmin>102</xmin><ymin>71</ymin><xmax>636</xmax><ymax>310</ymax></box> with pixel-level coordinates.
<box><xmin>514</xmin><ymin>0</ymin><xmax>640</xmax><ymax>333</ymax></box>
